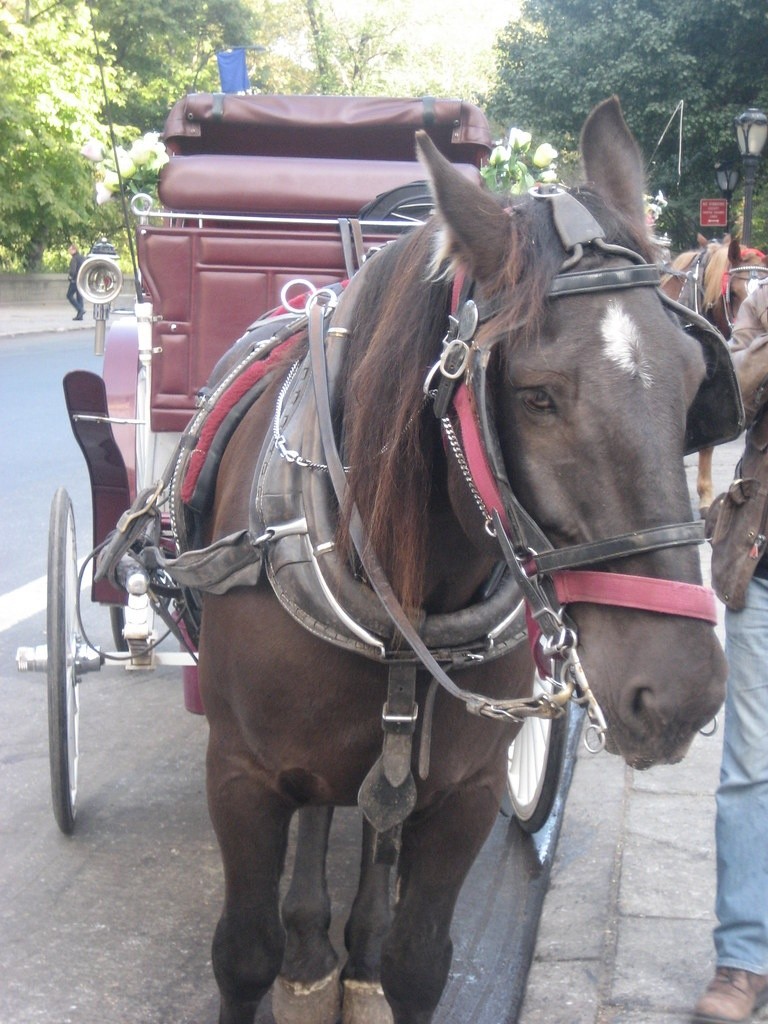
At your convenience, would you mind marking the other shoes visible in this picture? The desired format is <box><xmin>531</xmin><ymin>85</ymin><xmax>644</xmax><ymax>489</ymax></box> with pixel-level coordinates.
<box><xmin>77</xmin><ymin>310</ymin><xmax>86</xmax><ymax>318</ymax></box>
<box><xmin>73</xmin><ymin>315</ymin><xmax>82</xmax><ymax>320</ymax></box>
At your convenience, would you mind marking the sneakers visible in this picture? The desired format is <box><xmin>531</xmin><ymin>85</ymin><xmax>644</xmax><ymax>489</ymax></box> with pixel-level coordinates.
<box><xmin>694</xmin><ymin>967</ymin><xmax>768</xmax><ymax>1024</ymax></box>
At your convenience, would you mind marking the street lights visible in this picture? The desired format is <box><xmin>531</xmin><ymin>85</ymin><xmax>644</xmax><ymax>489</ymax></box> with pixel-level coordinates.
<box><xmin>714</xmin><ymin>162</ymin><xmax>740</xmax><ymax>233</ymax></box>
<box><xmin>733</xmin><ymin>107</ymin><xmax>768</xmax><ymax>246</ymax></box>
<box><xmin>191</xmin><ymin>45</ymin><xmax>265</xmax><ymax>94</ymax></box>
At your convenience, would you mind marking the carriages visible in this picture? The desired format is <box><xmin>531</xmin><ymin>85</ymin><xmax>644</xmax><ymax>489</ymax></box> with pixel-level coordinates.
<box><xmin>652</xmin><ymin>233</ymin><xmax>768</xmax><ymax>520</ymax></box>
<box><xmin>12</xmin><ymin>95</ymin><xmax>748</xmax><ymax>1023</ymax></box>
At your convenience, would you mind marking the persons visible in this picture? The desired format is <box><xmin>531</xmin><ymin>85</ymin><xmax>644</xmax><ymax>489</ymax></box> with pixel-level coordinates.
<box><xmin>66</xmin><ymin>245</ymin><xmax>87</xmax><ymax>320</ymax></box>
<box><xmin>692</xmin><ymin>283</ymin><xmax>768</xmax><ymax>1024</ymax></box>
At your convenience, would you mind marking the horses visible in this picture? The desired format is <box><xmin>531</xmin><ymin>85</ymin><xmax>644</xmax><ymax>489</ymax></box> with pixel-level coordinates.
<box><xmin>188</xmin><ymin>91</ymin><xmax>727</xmax><ymax>1024</ymax></box>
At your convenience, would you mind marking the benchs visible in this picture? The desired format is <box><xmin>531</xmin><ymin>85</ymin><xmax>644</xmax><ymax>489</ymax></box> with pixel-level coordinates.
<box><xmin>129</xmin><ymin>156</ymin><xmax>483</xmax><ymax>431</ymax></box>
